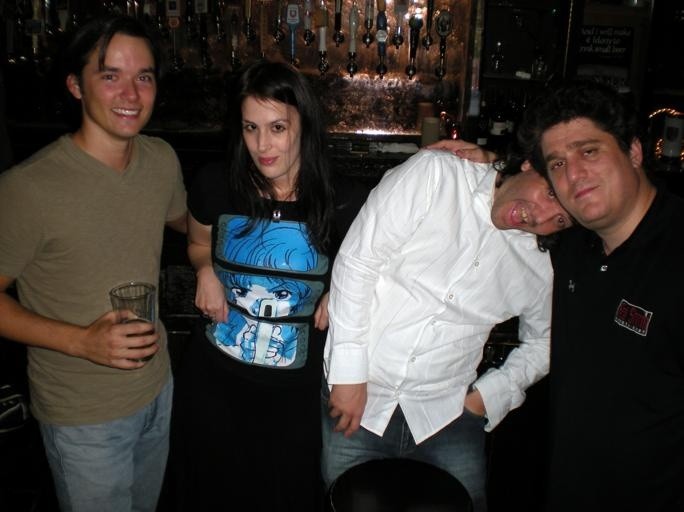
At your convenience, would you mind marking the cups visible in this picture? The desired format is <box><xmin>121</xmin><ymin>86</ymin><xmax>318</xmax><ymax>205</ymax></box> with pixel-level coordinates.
<box><xmin>109</xmin><ymin>282</ymin><xmax>156</xmax><ymax>362</ymax></box>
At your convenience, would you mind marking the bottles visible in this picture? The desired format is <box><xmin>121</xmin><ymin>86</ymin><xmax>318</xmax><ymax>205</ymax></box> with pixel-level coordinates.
<box><xmin>662</xmin><ymin>115</ymin><xmax>684</xmax><ymax>173</ymax></box>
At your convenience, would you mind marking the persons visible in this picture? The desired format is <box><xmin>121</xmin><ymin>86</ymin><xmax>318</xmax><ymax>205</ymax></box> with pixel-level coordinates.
<box><xmin>185</xmin><ymin>61</ymin><xmax>379</xmax><ymax>512</ymax></box>
<box><xmin>420</xmin><ymin>85</ymin><xmax>682</xmax><ymax>511</ymax></box>
<box><xmin>1</xmin><ymin>15</ymin><xmax>187</xmax><ymax>511</ymax></box>
<box><xmin>319</xmin><ymin>142</ymin><xmax>578</xmax><ymax>511</ymax></box>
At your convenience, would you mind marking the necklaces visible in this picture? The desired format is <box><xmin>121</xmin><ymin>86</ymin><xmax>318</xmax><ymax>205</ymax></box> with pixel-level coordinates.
<box><xmin>268</xmin><ymin>189</ymin><xmax>297</xmax><ymax>222</ymax></box>
